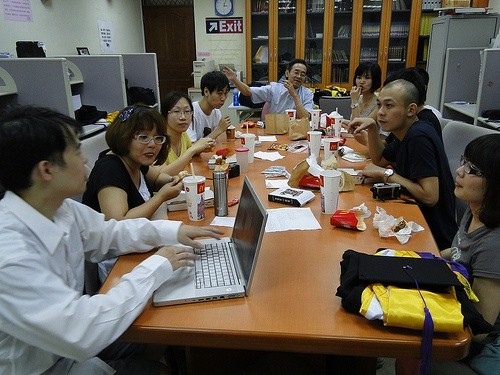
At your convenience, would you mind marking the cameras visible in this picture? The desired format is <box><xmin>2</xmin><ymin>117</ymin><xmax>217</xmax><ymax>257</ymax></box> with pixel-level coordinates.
<box><xmin>372</xmin><ymin>182</ymin><xmax>401</xmax><ymax>200</ymax></box>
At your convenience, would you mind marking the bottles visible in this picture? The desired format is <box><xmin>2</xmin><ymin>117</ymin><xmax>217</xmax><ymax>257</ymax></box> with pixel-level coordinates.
<box><xmin>233</xmin><ymin>88</ymin><xmax>239</xmax><ymax>106</ymax></box>
<box><xmin>213</xmin><ymin>171</ymin><xmax>228</xmax><ymax>217</ymax></box>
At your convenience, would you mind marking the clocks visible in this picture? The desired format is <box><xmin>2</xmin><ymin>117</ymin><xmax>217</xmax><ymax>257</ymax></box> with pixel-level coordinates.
<box><xmin>214</xmin><ymin>0</ymin><xmax>234</xmax><ymax>16</ymax></box>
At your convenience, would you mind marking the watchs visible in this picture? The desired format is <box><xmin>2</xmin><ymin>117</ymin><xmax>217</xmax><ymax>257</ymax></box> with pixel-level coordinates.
<box><xmin>189</xmin><ymin>147</ymin><xmax>199</xmax><ymax>160</ymax></box>
<box><xmin>384</xmin><ymin>169</ymin><xmax>394</xmax><ymax>183</ymax></box>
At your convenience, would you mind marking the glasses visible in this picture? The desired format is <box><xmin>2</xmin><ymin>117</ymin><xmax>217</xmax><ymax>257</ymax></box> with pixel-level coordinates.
<box><xmin>168</xmin><ymin>105</ymin><xmax>194</xmax><ymax>116</ymax></box>
<box><xmin>459</xmin><ymin>153</ymin><xmax>486</xmax><ymax>176</ymax></box>
<box><xmin>134</xmin><ymin>134</ymin><xmax>167</xmax><ymax>144</ymax></box>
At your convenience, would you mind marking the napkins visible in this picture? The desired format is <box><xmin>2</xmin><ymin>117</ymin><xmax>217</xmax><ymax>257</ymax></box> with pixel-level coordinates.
<box><xmin>210</xmin><ymin>215</ymin><xmax>235</xmax><ymax>227</ymax></box>
<box><xmin>265</xmin><ymin>207</ymin><xmax>322</xmax><ymax>232</ymax></box>
<box><xmin>254</xmin><ymin>151</ymin><xmax>286</xmax><ymax>162</ymax></box>
<box><xmin>258</xmin><ymin>136</ymin><xmax>277</xmax><ymax>141</ymax></box>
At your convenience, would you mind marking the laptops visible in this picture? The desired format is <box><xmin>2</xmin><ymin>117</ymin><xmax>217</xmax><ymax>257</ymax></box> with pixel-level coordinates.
<box><xmin>152</xmin><ymin>175</ymin><xmax>268</xmax><ymax>306</ymax></box>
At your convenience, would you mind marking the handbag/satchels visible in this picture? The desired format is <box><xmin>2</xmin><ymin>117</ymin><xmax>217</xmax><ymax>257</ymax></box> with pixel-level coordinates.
<box><xmin>312</xmin><ymin>88</ymin><xmax>332</xmax><ymax>105</ymax></box>
<box><xmin>238</xmin><ymin>81</ymin><xmax>268</xmax><ymax>108</ymax></box>
<box><xmin>128</xmin><ymin>86</ymin><xmax>156</xmax><ymax>105</ymax></box>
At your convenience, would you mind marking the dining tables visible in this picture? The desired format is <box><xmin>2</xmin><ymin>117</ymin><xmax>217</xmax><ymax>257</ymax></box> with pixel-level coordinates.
<box><xmin>98</xmin><ymin>125</ymin><xmax>472</xmax><ymax>375</ymax></box>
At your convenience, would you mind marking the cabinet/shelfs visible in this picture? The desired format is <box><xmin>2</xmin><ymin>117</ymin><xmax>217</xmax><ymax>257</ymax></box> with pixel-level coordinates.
<box><xmin>245</xmin><ymin>0</ymin><xmax>500</xmax><ymax>127</ymax></box>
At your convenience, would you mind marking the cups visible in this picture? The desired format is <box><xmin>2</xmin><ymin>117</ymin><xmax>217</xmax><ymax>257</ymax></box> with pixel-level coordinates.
<box><xmin>319</xmin><ymin>170</ymin><xmax>342</xmax><ymax>214</ymax></box>
<box><xmin>182</xmin><ymin>176</ymin><xmax>206</xmax><ymax>221</ymax></box>
<box><xmin>235</xmin><ymin>148</ymin><xmax>249</xmax><ymax>172</ymax></box>
<box><xmin>240</xmin><ymin>133</ymin><xmax>256</xmax><ymax>163</ymax></box>
<box><xmin>307</xmin><ymin>131</ymin><xmax>322</xmax><ymax>158</ymax></box>
<box><xmin>285</xmin><ymin>109</ymin><xmax>296</xmax><ymax>120</ymax></box>
<box><xmin>226</xmin><ymin>127</ymin><xmax>236</xmax><ymax>140</ymax></box>
<box><xmin>310</xmin><ymin>109</ymin><xmax>322</xmax><ymax>130</ymax></box>
<box><xmin>322</xmin><ymin>138</ymin><xmax>340</xmax><ymax>161</ymax></box>
<box><xmin>332</xmin><ymin>116</ymin><xmax>343</xmax><ymax>138</ymax></box>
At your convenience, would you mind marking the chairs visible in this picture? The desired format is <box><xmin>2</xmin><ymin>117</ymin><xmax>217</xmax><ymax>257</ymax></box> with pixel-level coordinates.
<box><xmin>442</xmin><ymin>121</ymin><xmax>500</xmax><ymax>181</ymax></box>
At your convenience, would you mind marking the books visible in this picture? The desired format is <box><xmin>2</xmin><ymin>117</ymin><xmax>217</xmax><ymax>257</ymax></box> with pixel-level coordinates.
<box><xmin>331</xmin><ymin>46</ymin><xmax>407</xmax><ymax>62</ymax></box>
<box><xmin>332</xmin><ymin>65</ymin><xmax>349</xmax><ymax>82</ymax></box>
<box><xmin>337</xmin><ymin>21</ymin><xmax>410</xmax><ymax>36</ymax></box>
<box><xmin>419</xmin><ymin>12</ymin><xmax>441</xmax><ymax>36</ymax></box>
<box><xmin>423</xmin><ymin>38</ymin><xmax>428</xmax><ymax>61</ymax></box>
<box><xmin>305</xmin><ymin>48</ymin><xmax>322</xmax><ymax>60</ymax></box>
<box><xmin>251</xmin><ymin>0</ymin><xmax>489</xmax><ymax>12</ymax></box>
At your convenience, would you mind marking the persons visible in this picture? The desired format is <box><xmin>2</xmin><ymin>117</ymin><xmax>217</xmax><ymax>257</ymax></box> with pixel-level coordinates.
<box><xmin>376</xmin><ymin>134</ymin><xmax>500</xmax><ymax>375</ymax></box>
<box><xmin>341</xmin><ymin>66</ymin><xmax>443</xmax><ymax>147</ymax></box>
<box><xmin>349</xmin><ymin>61</ymin><xmax>382</xmax><ymax>121</ymax></box>
<box><xmin>348</xmin><ymin>79</ymin><xmax>456</xmax><ymax>249</ymax></box>
<box><xmin>82</xmin><ymin>103</ymin><xmax>188</xmax><ymax>220</ymax></box>
<box><xmin>185</xmin><ymin>71</ymin><xmax>231</xmax><ymax>145</ymax></box>
<box><xmin>151</xmin><ymin>90</ymin><xmax>214</xmax><ymax>177</ymax></box>
<box><xmin>0</xmin><ymin>104</ymin><xmax>224</xmax><ymax>375</ymax></box>
<box><xmin>225</xmin><ymin>59</ymin><xmax>314</xmax><ymax>120</ymax></box>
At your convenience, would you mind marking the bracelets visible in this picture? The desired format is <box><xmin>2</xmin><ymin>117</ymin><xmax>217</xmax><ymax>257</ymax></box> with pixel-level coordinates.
<box><xmin>351</xmin><ymin>103</ymin><xmax>358</xmax><ymax>109</ymax></box>
